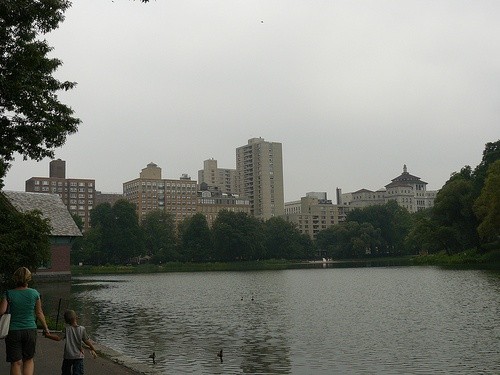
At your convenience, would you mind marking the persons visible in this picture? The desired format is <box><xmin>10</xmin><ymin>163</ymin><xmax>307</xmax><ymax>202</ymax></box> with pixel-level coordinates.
<box><xmin>42</xmin><ymin>310</ymin><xmax>97</xmax><ymax>375</ymax></box>
<box><xmin>0</xmin><ymin>267</ymin><xmax>50</xmax><ymax>375</ymax></box>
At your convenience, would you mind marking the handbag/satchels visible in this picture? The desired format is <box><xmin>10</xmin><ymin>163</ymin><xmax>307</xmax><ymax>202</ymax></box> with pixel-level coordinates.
<box><xmin>0</xmin><ymin>313</ymin><xmax>11</xmax><ymax>339</ymax></box>
